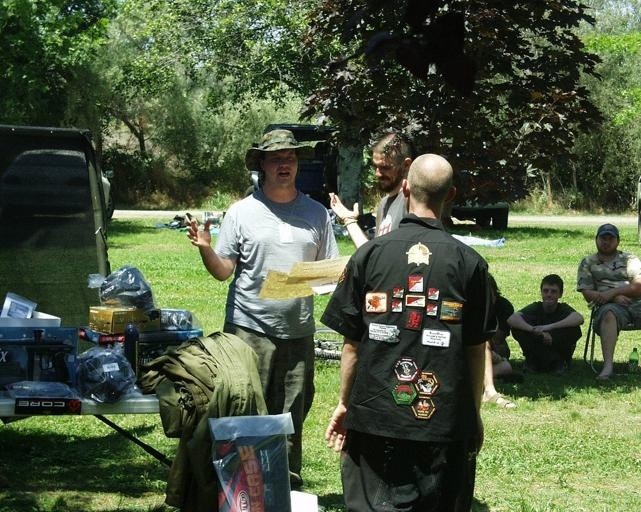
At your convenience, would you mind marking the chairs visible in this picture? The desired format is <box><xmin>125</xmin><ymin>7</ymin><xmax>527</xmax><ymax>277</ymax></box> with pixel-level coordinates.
<box><xmin>584</xmin><ymin>306</ymin><xmax>641</xmax><ymax>376</ymax></box>
<box><xmin>289</xmin><ymin>471</ymin><xmax>318</xmax><ymax>512</ymax></box>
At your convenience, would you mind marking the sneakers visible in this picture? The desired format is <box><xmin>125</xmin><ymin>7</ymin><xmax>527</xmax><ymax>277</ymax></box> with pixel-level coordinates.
<box><xmin>500</xmin><ymin>361</ymin><xmax>568</xmax><ymax>383</ymax></box>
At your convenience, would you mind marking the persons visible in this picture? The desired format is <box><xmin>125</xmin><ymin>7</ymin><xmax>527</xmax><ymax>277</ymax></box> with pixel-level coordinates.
<box><xmin>506</xmin><ymin>274</ymin><xmax>584</xmax><ymax>374</ymax></box>
<box><xmin>185</xmin><ymin>128</ymin><xmax>347</xmax><ymax>492</ymax></box>
<box><xmin>483</xmin><ymin>270</ymin><xmax>519</xmax><ymax>408</ymax></box>
<box><xmin>319</xmin><ymin>153</ymin><xmax>497</xmax><ymax>511</ymax></box>
<box><xmin>577</xmin><ymin>222</ymin><xmax>641</xmax><ymax>382</ymax></box>
<box><xmin>327</xmin><ymin>132</ymin><xmax>413</xmax><ymax>250</ymax></box>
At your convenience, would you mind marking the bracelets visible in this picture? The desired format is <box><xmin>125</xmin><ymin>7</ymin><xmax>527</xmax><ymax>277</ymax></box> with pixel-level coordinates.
<box><xmin>340</xmin><ymin>215</ymin><xmax>360</xmax><ymax>228</ymax></box>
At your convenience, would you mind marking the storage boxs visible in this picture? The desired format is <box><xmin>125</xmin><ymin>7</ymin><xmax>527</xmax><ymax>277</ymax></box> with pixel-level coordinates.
<box><xmin>0</xmin><ymin>305</ymin><xmax>203</xmax><ymax>414</ymax></box>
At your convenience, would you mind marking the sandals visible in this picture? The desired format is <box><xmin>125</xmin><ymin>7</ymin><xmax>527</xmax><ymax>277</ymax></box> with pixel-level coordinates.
<box><xmin>482</xmin><ymin>391</ymin><xmax>517</xmax><ymax>411</ymax></box>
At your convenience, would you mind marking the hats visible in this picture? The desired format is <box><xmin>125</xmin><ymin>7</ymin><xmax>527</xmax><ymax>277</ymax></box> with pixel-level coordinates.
<box><xmin>596</xmin><ymin>224</ymin><xmax>619</xmax><ymax>240</ymax></box>
<box><xmin>244</xmin><ymin>129</ymin><xmax>328</xmax><ymax>173</ymax></box>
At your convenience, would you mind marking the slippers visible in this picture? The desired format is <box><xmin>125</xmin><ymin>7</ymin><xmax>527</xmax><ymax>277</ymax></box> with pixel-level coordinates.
<box><xmin>594</xmin><ymin>367</ymin><xmax>613</xmax><ymax>382</ymax></box>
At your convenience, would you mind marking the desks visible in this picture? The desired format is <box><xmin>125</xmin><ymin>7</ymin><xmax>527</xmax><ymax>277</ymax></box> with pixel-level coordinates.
<box><xmin>0</xmin><ymin>394</ymin><xmax>177</xmax><ymax>468</ymax></box>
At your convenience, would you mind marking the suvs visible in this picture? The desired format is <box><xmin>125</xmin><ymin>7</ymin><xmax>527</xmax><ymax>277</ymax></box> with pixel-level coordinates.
<box><xmin>248</xmin><ymin>122</ymin><xmax>339</xmax><ymax>216</ymax></box>
<box><xmin>422</xmin><ymin>139</ymin><xmax>527</xmax><ymax>228</ymax></box>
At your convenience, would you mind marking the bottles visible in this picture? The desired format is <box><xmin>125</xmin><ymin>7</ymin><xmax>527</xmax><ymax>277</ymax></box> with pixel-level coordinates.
<box><xmin>123</xmin><ymin>322</ymin><xmax>140</xmax><ymax>371</ymax></box>
